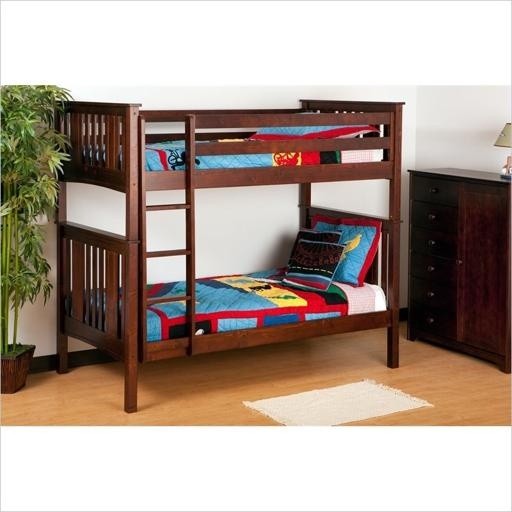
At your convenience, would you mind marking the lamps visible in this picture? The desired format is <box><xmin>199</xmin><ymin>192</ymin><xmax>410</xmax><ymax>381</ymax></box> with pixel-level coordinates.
<box><xmin>493</xmin><ymin>123</ymin><xmax>512</xmax><ymax>179</ymax></box>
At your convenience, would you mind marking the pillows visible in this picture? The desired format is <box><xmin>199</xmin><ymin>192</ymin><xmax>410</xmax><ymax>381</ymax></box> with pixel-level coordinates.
<box><xmin>279</xmin><ymin>214</ymin><xmax>383</xmax><ymax>293</ymax></box>
<box><xmin>249</xmin><ymin>111</ymin><xmax>381</xmax><ymax>146</ymax></box>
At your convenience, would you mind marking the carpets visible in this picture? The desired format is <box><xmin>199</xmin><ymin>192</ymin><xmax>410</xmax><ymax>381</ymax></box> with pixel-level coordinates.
<box><xmin>242</xmin><ymin>377</ymin><xmax>433</xmax><ymax>426</ymax></box>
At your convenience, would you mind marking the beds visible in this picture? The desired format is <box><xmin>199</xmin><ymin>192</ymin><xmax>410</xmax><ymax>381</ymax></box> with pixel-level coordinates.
<box><xmin>54</xmin><ymin>99</ymin><xmax>406</xmax><ymax>413</ymax></box>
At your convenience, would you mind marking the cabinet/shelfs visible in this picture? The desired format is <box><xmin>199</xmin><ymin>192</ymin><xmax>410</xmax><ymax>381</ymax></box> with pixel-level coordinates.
<box><xmin>405</xmin><ymin>169</ymin><xmax>511</xmax><ymax>374</ymax></box>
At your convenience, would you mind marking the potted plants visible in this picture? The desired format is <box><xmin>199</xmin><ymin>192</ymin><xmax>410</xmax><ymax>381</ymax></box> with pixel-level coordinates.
<box><xmin>1</xmin><ymin>85</ymin><xmax>75</xmax><ymax>395</ymax></box>
